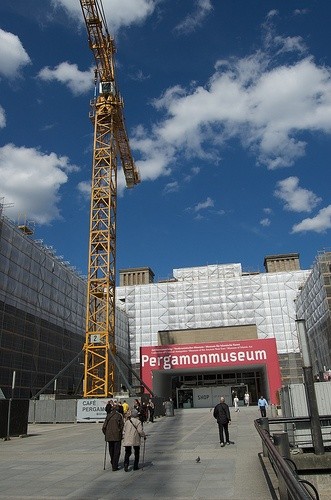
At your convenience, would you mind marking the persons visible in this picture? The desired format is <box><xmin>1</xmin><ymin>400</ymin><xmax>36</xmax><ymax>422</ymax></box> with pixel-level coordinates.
<box><xmin>102</xmin><ymin>407</ymin><xmax>124</xmax><ymax>471</ymax></box>
<box><xmin>167</xmin><ymin>395</ymin><xmax>175</xmax><ymax>416</ymax></box>
<box><xmin>122</xmin><ymin>410</ymin><xmax>150</xmax><ymax>472</ymax></box>
<box><xmin>142</xmin><ymin>396</ymin><xmax>163</xmax><ymax>424</ymax></box>
<box><xmin>134</xmin><ymin>399</ymin><xmax>141</xmax><ymax>412</ymax></box>
<box><xmin>105</xmin><ymin>400</ymin><xmax>129</xmax><ymax>415</ymax></box>
<box><xmin>179</xmin><ymin>394</ymin><xmax>186</xmax><ymax>408</ymax></box>
<box><xmin>213</xmin><ymin>397</ymin><xmax>231</xmax><ymax>447</ymax></box>
<box><xmin>234</xmin><ymin>396</ymin><xmax>239</xmax><ymax>412</ymax></box>
<box><xmin>244</xmin><ymin>392</ymin><xmax>249</xmax><ymax>407</ymax></box>
<box><xmin>258</xmin><ymin>396</ymin><xmax>268</xmax><ymax>418</ymax></box>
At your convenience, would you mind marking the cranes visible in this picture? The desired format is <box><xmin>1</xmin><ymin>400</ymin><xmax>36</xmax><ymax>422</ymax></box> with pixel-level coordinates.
<box><xmin>80</xmin><ymin>0</ymin><xmax>142</xmax><ymax>399</ymax></box>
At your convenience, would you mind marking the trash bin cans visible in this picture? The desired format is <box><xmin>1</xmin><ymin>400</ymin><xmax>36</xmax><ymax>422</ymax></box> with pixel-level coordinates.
<box><xmin>164</xmin><ymin>401</ymin><xmax>174</xmax><ymax>416</ymax></box>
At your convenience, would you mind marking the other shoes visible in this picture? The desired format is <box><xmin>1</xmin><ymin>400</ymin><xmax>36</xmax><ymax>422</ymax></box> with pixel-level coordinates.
<box><xmin>112</xmin><ymin>468</ymin><xmax>116</xmax><ymax>471</ymax></box>
<box><xmin>221</xmin><ymin>442</ymin><xmax>224</xmax><ymax>447</ymax></box>
<box><xmin>226</xmin><ymin>442</ymin><xmax>230</xmax><ymax>445</ymax></box>
<box><xmin>133</xmin><ymin>467</ymin><xmax>140</xmax><ymax>470</ymax></box>
<box><xmin>116</xmin><ymin>468</ymin><xmax>120</xmax><ymax>470</ymax></box>
<box><xmin>124</xmin><ymin>467</ymin><xmax>128</xmax><ymax>472</ymax></box>
